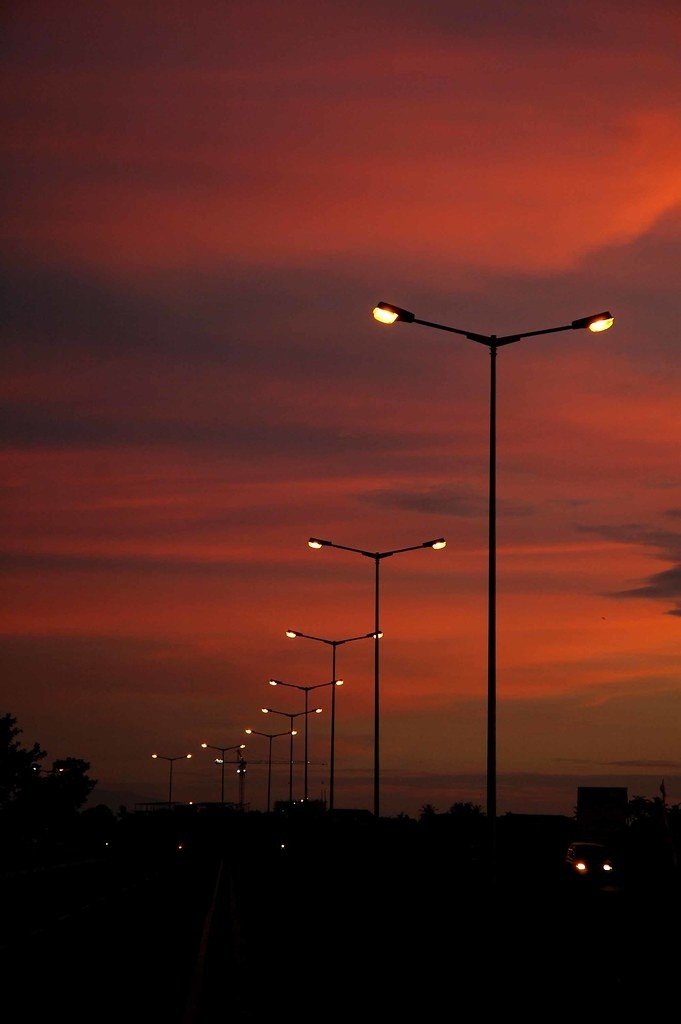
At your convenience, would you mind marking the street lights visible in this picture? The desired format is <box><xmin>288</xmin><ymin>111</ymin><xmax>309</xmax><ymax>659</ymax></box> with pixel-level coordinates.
<box><xmin>245</xmin><ymin>727</ymin><xmax>296</xmax><ymax>812</ymax></box>
<box><xmin>150</xmin><ymin>753</ymin><xmax>192</xmax><ymax>809</ymax></box>
<box><xmin>199</xmin><ymin>741</ymin><xmax>246</xmax><ymax>805</ymax></box>
<box><xmin>262</xmin><ymin>705</ymin><xmax>323</xmax><ymax>807</ymax></box>
<box><xmin>283</xmin><ymin>630</ymin><xmax>384</xmax><ymax>811</ymax></box>
<box><xmin>371</xmin><ymin>301</ymin><xmax>614</xmax><ymax>816</ymax></box>
<box><xmin>267</xmin><ymin>677</ymin><xmax>345</xmax><ymax>807</ymax></box>
<box><xmin>306</xmin><ymin>537</ymin><xmax>446</xmax><ymax>822</ymax></box>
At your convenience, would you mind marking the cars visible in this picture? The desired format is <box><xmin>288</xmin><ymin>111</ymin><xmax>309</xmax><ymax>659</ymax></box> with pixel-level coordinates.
<box><xmin>566</xmin><ymin>841</ymin><xmax>613</xmax><ymax>882</ymax></box>
